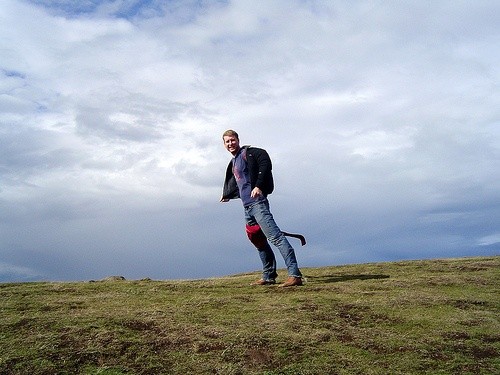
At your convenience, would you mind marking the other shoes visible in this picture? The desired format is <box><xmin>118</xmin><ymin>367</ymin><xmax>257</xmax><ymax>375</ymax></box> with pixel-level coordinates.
<box><xmin>250</xmin><ymin>279</ymin><xmax>268</xmax><ymax>286</ymax></box>
<box><xmin>278</xmin><ymin>276</ymin><xmax>303</xmax><ymax>288</ymax></box>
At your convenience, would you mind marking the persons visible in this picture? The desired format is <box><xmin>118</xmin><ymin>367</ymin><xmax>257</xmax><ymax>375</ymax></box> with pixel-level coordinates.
<box><xmin>220</xmin><ymin>130</ymin><xmax>303</xmax><ymax>287</ymax></box>
